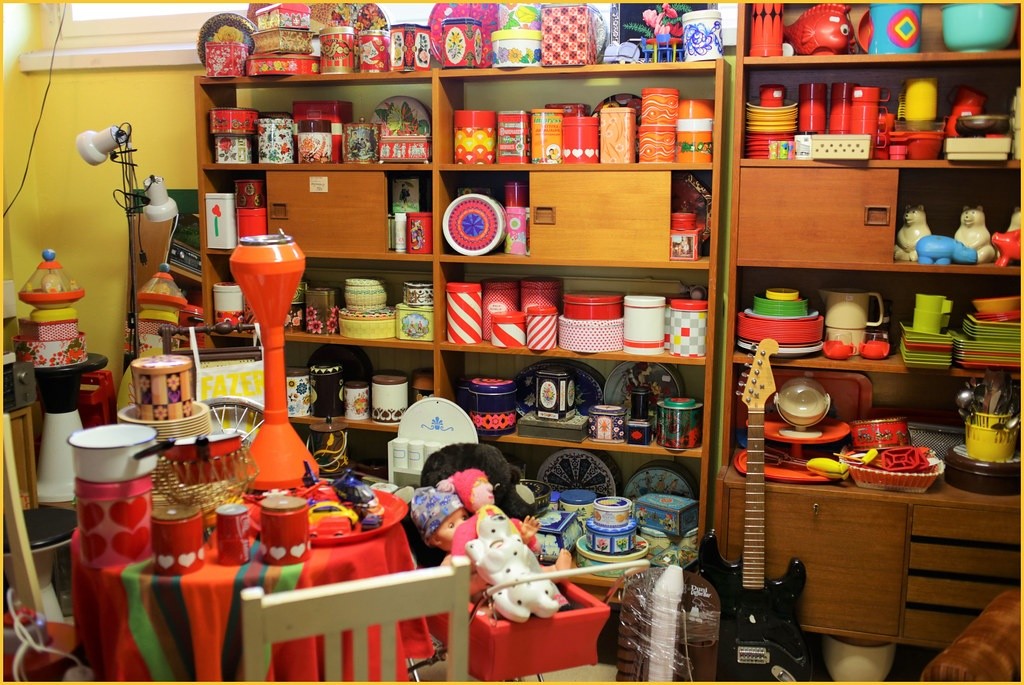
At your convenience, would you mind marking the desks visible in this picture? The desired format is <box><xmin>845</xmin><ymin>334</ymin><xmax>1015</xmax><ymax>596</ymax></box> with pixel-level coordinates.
<box><xmin>36</xmin><ymin>351</ymin><xmax>108</xmax><ymax>502</ymax></box>
<box><xmin>74</xmin><ymin>482</ymin><xmax>433</xmax><ymax>681</ymax></box>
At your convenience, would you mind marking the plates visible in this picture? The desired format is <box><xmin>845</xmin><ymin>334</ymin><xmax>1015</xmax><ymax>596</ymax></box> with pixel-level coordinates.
<box><xmin>745</xmin><ymin>98</ymin><xmax>799</xmax><ymax>160</ymax></box>
<box><xmin>736</xmin><ymin>292</ymin><xmax>825</xmax><ymax>359</ymax></box>
<box><xmin>897</xmin><ymin>312</ymin><xmax>1020</xmax><ymax>373</ymax></box>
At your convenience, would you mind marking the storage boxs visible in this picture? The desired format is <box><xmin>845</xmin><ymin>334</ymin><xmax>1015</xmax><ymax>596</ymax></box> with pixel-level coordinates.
<box><xmin>518</xmin><ymin>410</ymin><xmax>592</xmax><ymax>443</ymax></box>
<box><xmin>631</xmin><ymin>491</ymin><xmax>699</xmax><ymax>554</ymax></box>
<box><xmin>669</xmin><ymin>228</ymin><xmax>703</xmax><ymax>261</ymax></box>
<box><xmin>250</xmin><ymin>3</ymin><xmax>316</xmax><ymax>55</ymax></box>
<box><xmin>377</xmin><ymin>136</ymin><xmax>433</xmax><ymax>164</ymax></box>
<box><xmin>811</xmin><ymin>134</ymin><xmax>871</xmax><ymax>161</ymax></box>
<box><xmin>204</xmin><ymin>41</ymin><xmax>250</xmax><ymax>78</ymax></box>
<box><xmin>426</xmin><ymin>577</ymin><xmax>611</xmax><ymax>682</ymax></box>
<box><xmin>942</xmin><ymin>138</ymin><xmax>1012</xmax><ymax>161</ymax></box>
<box><xmin>539</xmin><ymin>2</ymin><xmax>598</xmax><ymax>65</ymax></box>
<box><xmin>292</xmin><ymin>100</ymin><xmax>353</xmax><ymax>126</ymax></box>
<box><xmin>535</xmin><ymin>510</ymin><xmax>583</xmax><ymax>564</ymax></box>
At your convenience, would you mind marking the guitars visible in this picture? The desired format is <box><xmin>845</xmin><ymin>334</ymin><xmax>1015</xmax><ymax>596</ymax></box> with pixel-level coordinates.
<box><xmin>695</xmin><ymin>337</ymin><xmax>812</xmax><ymax>682</ymax></box>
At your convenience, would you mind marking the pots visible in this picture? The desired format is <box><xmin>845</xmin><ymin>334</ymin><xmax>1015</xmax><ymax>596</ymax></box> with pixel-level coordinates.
<box><xmin>165</xmin><ymin>432</ymin><xmax>241</xmax><ymax>464</ymax></box>
<box><xmin>66</xmin><ymin>423</ymin><xmax>176</xmax><ymax>484</ymax></box>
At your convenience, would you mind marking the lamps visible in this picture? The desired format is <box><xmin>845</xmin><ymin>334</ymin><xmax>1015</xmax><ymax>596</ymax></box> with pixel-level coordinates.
<box><xmin>76</xmin><ymin>117</ymin><xmax>179</xmax><ymax>379</ymax></box>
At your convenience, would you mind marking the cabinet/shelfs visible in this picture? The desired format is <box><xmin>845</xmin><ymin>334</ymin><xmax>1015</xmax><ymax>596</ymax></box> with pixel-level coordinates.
<box><xmin>710</xmin><ymin>3</ymin><xmax>1021</xmax><ymax>649</ymax></box>
<box><xmin>191</xmin><ymin>59</ymin><xmax>725</xmax><ymax>544</ymax></box>
<box><xmin>126</xmin><ymin>214</ymin><xmax>203</xmax><ymax>323</ymax></box>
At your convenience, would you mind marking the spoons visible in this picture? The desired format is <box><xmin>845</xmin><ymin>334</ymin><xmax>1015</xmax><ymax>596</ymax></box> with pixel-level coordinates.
<box><xmin>959</xmin><ymin>369</ymin><xmax>1021</xmax><ymax>433</ymax></box>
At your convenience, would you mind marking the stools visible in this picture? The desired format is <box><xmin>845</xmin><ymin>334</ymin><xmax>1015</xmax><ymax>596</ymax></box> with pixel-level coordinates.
<box><xmin>2</xmin><ymin>508</ymin><xmax>78</xmax><ymax>627</ymax></box>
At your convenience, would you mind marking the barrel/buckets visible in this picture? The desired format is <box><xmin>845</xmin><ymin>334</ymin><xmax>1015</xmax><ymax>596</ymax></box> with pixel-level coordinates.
<box><xmin>150</xmin><ymin>507</ymin><xmax>205</xmax><ymax>576</ymax></box>
<box><xmin>72</xmin><ymin>476</ymin><xmax>152</xmax><ymax>567</ymax></box>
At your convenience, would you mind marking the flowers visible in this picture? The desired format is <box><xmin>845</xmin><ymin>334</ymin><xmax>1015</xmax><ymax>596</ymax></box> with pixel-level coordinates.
<box><xmin>620</xmin><ymin>3</ymin><xmax>693</xmax><ymax>38</ymax></box>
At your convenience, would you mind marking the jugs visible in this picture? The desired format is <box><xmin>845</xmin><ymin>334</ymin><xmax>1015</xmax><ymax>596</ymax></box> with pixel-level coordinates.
<box><xmin>818</xmin><ymin>286</ymin><xmax>884</xmax><ymax>356</ymax></box>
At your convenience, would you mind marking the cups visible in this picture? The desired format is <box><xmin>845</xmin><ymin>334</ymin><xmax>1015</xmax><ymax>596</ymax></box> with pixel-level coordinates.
<box><xmin>506</xmin><ymin>207</ymin><xmax>527</xmax><ymax>255</ymax></box>
<box><xmin>286</xmin><ymin>362</ymin><xmax>408</xmax><ymax>426</ymax></box>
<box><xmin>799</xmin><ymin>82</ymin><xmax>907</xmax><ymax>162</ymax></box>
<box><xmin>216</xmin><ymin>503</ymin><xmax>250</xmax><ymax>567</ymax></box>
<box><xmin>446</xmin><ymin>278</ymin><xmax>561</xmax><ymax>351</ymax></box>
<box><xmin>858</xmin><ymin>330</ymin><xmax>890</xmax><ymax>360</ymax></box>
<box><xmin>260</xmin><ymin>496</ymin><xmax>313</xmax><ymax>567</ymax></box>
<box><xmin>941</xmin><ymin>83</ymin><xmax>985</xmax><ymax>137</ymax></box>
<box><xmin>452</xmin><ymin>86</ymin><xmax>713</xmax><ymax>168</ymax></box>
<box><xmin>319</xmin><ymin>25</ymin><xmax>390</xmax><ymax>74</ymax></box>
<box><xmin>682</xmin><ymin>9</ymin><xmax>723</xmax><ymax>62</ymax></box>
<box><xmin>759</xmin><ymin>84</ymin><xmax>784</xmax><ymax>107</ymax></box>
<box><xmin>913</xmin><ymin>292</ymin><xmax>954</xmax><ymax>334</ymax></box>
<box><xmin>406</xmin><ymin>212</ymin><xmax>433</xmax><ymax>255</ymax></box>
<box><xmin>504</xmin><ymin>179</ymin><xmax>528</xmax><ymax>206</ymax></box>
<box><xmin>823</xmin><ymin>330</ymin><xmax>857</xmax><ymax>361</ymax></box>
<box><xmin>868</xmin><ymin>3</ymin><xmax>923</xmax><ymax>55</ymax></box>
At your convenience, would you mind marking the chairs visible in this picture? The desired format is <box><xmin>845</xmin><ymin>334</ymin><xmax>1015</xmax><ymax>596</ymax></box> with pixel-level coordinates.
<box><xmin>239</xmin><ymin>553</ymin><xmax>471</xmax><ymax>682</ymax></box>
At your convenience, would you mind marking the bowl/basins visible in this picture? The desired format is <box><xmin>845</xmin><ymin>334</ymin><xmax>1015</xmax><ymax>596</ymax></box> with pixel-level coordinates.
<box><xmin>941</xmin><ymin>4</ymin><xmax>1019</xmax><ymax>53</ymax></box>
<box><xmin>971</xmin><ymin>297</ymin><xmax>1020</xmax><ymax>313</ymax></box>
<box><xmin>117</xmin><ymin>404</ymin><xmax>220</xmax><ymax>519</ymax></box>
<box><xmin>955</xmin><ymin>115</ymin><xmax>1010</xmax><ymax>138</ymax></box>
<box><xmin>821</xmin><ymin>635</ymin><xmax>896</xmax><ymax>682</ymax></box>
<box><xmin>889</xmin><ymin>120</ymin><xmax>945</xmax><ymax>160</ymax></box>
